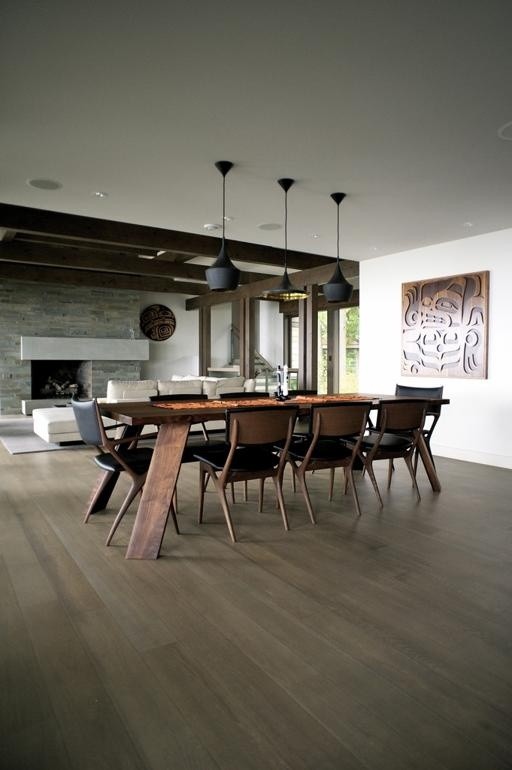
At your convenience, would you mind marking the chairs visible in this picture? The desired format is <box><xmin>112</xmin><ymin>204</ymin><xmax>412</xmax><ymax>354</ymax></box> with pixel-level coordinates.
<box><xmin>147</xmin><ymin>390</ymin><xmax>317</xmax><ymax>403</ymax></box>
<box><xmin>387</xmin><ymin>384</ymin><xmax>443</xmax><ymax>490</ymax></box>
<box><xmin>70</xmin><ymin>391</ymin><xmax>180</xmax><ymax>547</ymax></box>
<box><xmin>265</xmin><ymin>368</ymin><xmax>300</xmax><ymax>395</ymax></box>
<box><xmin>192</xmin><ymin>405</ymin><xmax>300</xmax><ymax>543</ymax></box>
<box><xmin>342</xmin><ymin>399</ymin><xmax>429</xmax><ymax>509</ymax></box>
<box><xmin>274</xmin><ymin>401</ymin><xmax>373</xmax><ymax>525</ymax></box>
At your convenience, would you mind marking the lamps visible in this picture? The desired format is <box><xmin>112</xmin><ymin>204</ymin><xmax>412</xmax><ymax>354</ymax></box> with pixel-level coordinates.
<box><xmin>323</xmin><ymin>192</ymin><xmax>353</xmax><ymax>304</ymax></box>
<box><xmin>205</xmin><ymin>160</ymin><xmax>240</xmax><ymax>292</ymax></box>
<box><xmin>261</xmin><ymin>178</ymin><xmax>311</xmax><ymax>302</ymax></box>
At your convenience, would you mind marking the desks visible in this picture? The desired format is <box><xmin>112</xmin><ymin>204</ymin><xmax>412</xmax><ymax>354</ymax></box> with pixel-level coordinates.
<box><xmin>84</xmin><ymin>393</ymin><xmax>450</xmax><ymax>514</ymax></box>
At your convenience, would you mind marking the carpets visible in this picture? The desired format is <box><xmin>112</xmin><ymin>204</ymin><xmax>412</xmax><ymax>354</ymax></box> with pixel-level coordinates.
<box><xmin>0</xmin><ymin>417</ymin><xmax>155</xmax><ymax>455</ymax></box>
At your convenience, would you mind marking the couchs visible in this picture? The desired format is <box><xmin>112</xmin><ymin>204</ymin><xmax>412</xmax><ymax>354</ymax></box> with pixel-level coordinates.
<box><xmin>32</xmin><ymin>374</ymin><xmax>255</xmax><ymax>447</ymax></box>
